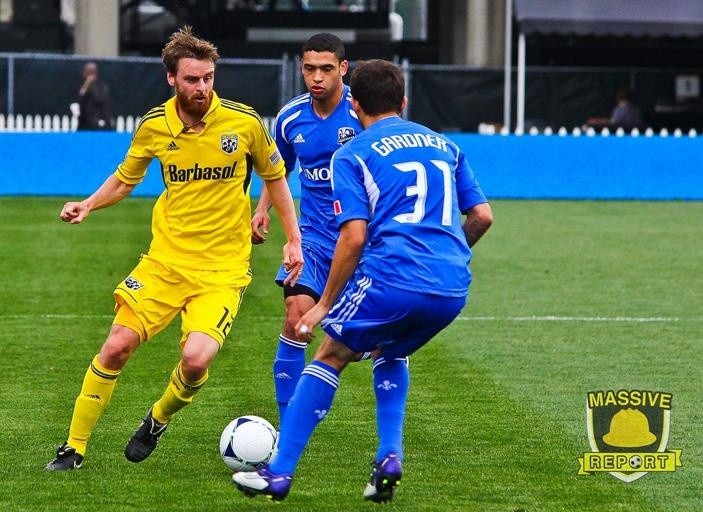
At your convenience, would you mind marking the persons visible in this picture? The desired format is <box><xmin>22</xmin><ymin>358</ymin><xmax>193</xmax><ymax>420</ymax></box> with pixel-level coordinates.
<box><xmin>608</xmin><ymin>85</ymin><xmax>643</xmax><ymax>134</ymax></box>
<box><xmin>76</xmin><ymin>63</ymin><xmax>115</xmax><ymax>131</ymax></box>
<box><xmin>223</xmin><ymin>140</ymin><xmax>236</xmax><ymax>153</ymax></box>
<box><xmin>251</xmin><ymin>32</ymin><xmax>372</xmax><ymax>432</ymax></box>
<box><xmin>46</xmin><ymin>25</ymin><xmax>304</xmax><ymax>470</ymax></box>
<box><xmin>231</xmin><ymin>60</ymin><xmax>493</xmax><ymax>504</ymax></box>
<box><xmin>339</xmin><ymin>128</ymin><xmax>353</xmax><ymax>145</ymax></box>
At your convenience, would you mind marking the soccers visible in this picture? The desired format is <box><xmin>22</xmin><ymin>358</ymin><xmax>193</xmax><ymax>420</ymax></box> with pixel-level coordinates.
<box><xmin>219</xmin><ymin>414</ymin><xmax>278</xmax><ymax>473</ymax></box>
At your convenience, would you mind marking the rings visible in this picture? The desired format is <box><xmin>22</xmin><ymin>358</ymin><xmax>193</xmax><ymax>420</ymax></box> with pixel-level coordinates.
<box><xmin>300</xmin><ymin>325</ymin><xmax>308</xmax><ymax>333</ymax></box>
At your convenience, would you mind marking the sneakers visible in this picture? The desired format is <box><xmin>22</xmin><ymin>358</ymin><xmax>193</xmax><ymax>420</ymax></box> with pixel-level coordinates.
<box><xmin>124</xmin><ymin>404</ymin><xmax>171</xmax><ymax>463</ymax></box>
<box><xmin>231</xmin><ymin>464</ymin><xmax>292</xmax><ymax>505</ymax></box>
<box><xmin>46</xmin><ymin>446</ymin><xmax>82</xmax><ymax>471</ymax></box>
<box><xmin>363</xmin><ymin>452</ymin><xmax>402</xmax><ymax>504</ymax></box>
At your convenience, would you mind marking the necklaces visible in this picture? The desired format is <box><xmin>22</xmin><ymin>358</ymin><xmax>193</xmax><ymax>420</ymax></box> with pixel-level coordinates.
<box><xmin>181</xmin><ymin>120</ymin><xmax>202</xmax><ymax>133</ymax></box>
